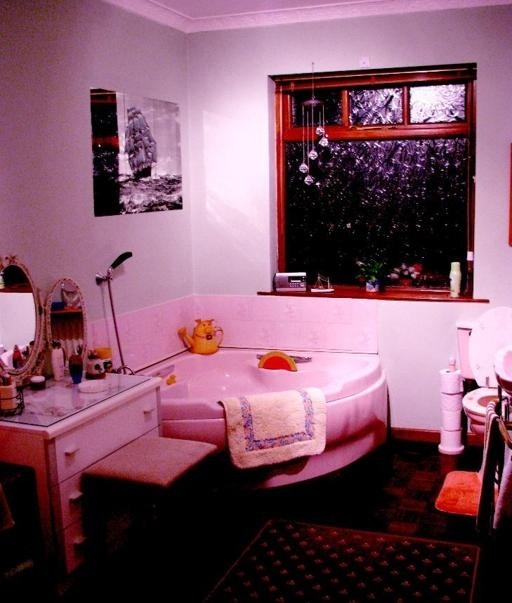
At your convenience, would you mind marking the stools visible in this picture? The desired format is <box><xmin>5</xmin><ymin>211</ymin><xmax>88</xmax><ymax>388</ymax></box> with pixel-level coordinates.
<box><xmin>78</xmin><ymin>433</ymin><xmax>220</xmax><ymax>574</ymax></box>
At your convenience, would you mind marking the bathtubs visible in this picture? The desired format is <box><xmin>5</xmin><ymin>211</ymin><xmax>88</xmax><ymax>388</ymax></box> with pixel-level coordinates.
<box><xmin>131</xmin><ymin>349</ymin><xmax>387</xmax><ymax>488</ymax></box>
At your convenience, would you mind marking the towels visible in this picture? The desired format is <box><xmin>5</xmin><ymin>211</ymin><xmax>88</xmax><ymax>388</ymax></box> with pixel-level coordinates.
<box><xmin>475</xmin><ymin>395</ymin><xmax>497</xmax><ymax>530</ymax></box>
<box><xmin>217</xmin><ymin>387</ymin><xmax>327</xmax><ymax>469</ymax></box>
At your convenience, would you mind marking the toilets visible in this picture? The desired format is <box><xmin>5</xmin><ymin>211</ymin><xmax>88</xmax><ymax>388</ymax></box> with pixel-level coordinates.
<box><xmin>461</xmin><ymin>304</ymin><xmax>512</xmax><ymax>444</ymax></box>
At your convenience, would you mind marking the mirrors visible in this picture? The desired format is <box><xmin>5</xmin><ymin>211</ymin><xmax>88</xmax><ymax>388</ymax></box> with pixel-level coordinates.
<box><xmin>0</xmin><ymin>251</ymin><xmax>45</xmax><ymax>384</ymax></box>
<box><xmin>44</xmin><ymin>278</ymin><xmax>90</xmax><ymax>368</ymax></box>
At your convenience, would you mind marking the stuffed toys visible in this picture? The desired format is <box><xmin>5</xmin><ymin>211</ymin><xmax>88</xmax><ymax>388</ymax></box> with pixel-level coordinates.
<box><xmin>177</xmin><ymin>318</ymin><xmax>225</xmax><ymax>355</ymax></box>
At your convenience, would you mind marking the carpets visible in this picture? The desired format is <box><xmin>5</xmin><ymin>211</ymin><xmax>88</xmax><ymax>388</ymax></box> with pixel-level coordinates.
<box><xmin>198</xmin><ymin>516</ymin><xmax>482</xmax><ymax>603</ymax></box>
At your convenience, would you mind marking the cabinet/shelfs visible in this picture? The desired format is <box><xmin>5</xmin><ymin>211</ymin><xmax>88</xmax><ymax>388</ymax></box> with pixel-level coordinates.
<box><xmin>1</xmin><ymin>369</ymin><xmax>165</xmax><ymax>575</ymax></box>
